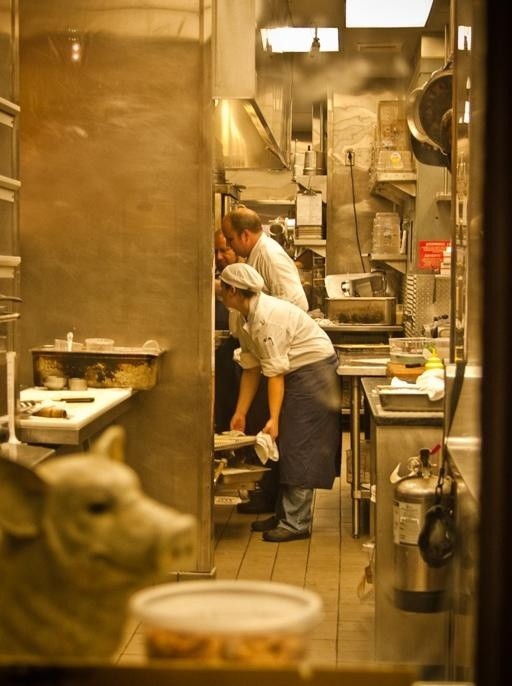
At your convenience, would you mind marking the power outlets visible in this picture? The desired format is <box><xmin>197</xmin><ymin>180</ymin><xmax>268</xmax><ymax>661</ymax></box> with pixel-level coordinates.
<box><xmin>345</xmin><ymin>152</ymin><xmax>355</xmax><ymax>165</ymax></box>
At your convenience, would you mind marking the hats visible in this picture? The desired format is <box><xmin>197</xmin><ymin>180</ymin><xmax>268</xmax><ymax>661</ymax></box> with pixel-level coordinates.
<box><xmin>219</xmin><ymin>262</ymin><xmax>264</xmax><ymax>294</ymax></box>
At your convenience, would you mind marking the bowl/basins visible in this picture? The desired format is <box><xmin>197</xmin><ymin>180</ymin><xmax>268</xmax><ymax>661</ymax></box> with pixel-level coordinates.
<box><xmin>41</xmin><ymin>338</ymin><xmax>118</xmax><ymax>350</ymax></box>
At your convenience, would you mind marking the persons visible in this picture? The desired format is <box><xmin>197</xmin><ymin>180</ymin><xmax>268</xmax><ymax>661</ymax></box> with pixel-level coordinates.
<box><xmin>219</xmin><ymin>208</ymin><xmax>310</xmax><ymax>513</ymax></box>
<box><xmin>214</xmin><ymin>229</ymin><xmax>264</xmax><ymax>480</ymax></box>
<box><xmin>219</xmin><ymin>263</ymin><xmax>343</xmax><ymax>541</ymax></box>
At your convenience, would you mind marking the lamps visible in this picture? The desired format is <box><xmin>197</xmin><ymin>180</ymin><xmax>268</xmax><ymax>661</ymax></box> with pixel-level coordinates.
<box><xmin>309</xmin><ymin>27</ymin><xmax>320</xmax><ymax>57</ymax></box>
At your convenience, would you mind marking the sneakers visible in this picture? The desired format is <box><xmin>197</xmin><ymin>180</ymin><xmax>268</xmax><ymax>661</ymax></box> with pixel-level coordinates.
<box><xmin>263</xmin><ymin>526</ymin><xmax>310</xmax><ymax>542</ymax></box>
<box><xmin>237</xmin><ymin>495</ymin><xmax>275</xmax><ymax>513</ymax></box>
<box><xmin>251</xmin><ymin>516</ymin><xmax>279</xmax><ymax>531</ymax></box>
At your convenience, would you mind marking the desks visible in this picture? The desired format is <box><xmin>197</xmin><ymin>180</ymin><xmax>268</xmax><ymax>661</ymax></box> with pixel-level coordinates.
<box><xmin>1</xmin><ymin>384</ymin><xmax>140</xmax><ymax>451</ymax></box>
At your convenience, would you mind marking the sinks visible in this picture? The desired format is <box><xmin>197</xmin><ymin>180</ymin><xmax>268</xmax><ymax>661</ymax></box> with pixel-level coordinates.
<box><xmin>378</xmin><ymin>391</ymin><xmax>443</xmax><ymax>411</ymax></box>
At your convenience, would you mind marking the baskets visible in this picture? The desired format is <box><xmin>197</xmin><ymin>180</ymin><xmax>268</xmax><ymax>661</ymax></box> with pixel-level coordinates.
<box><xmin>388</xmin><ymin>336</ymin><xmax>436</xmax><ymax>365</ymax></box>
